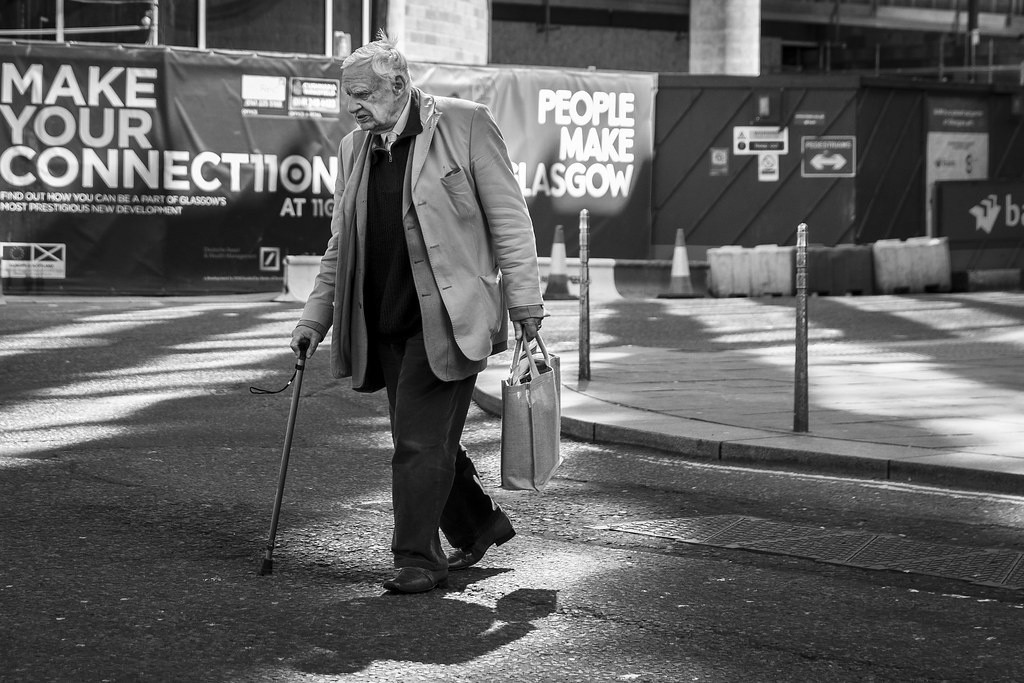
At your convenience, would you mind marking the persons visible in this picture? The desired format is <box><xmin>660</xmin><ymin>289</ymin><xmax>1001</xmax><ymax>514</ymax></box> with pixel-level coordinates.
<box><xmin>290</xmin><ymin>26</ymin><xmax>543</xmax><ymax>593</ymax></box>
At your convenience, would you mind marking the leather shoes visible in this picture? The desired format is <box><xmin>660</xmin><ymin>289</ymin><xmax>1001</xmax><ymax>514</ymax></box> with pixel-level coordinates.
<box><xmin>383</xmin><ymin>567</ymin><xmax>449</xmax><ymax>593</ymax></box>
<box><xmin>448</xmin><ymin>510</ymin><xmax>517</xmax><ymax>570</ymax></box>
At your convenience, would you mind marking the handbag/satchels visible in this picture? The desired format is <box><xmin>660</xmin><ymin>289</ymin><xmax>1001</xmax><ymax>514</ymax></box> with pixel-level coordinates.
<box><xmin>497</xmin><ymin>327</ymin><xmax>564</xmax><ymax>492</ymax></box>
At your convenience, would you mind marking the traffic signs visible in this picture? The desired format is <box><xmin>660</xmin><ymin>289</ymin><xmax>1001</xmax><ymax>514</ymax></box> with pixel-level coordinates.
<box><xmin>800</xmin><ymin>136</ymin><xmax>857</xmax><ymax>178</ymax></box>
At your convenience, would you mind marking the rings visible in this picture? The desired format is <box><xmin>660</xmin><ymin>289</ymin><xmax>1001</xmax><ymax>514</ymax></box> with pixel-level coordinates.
<box><xmin>538</xmin><ymin>324</ymin><xmax>542</xmax><ymax>328</ymax></box>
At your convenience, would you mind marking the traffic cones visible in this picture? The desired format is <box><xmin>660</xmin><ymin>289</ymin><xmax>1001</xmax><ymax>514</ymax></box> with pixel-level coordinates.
<box><xmin>541</xmin><ymin>224</ymin><xmax>579</xmax><ymax>300</ymax></box>
<box><xmin>656</xmin><ymin>228</ymin><xmax>705</xmax><ymax>299</ymax></box>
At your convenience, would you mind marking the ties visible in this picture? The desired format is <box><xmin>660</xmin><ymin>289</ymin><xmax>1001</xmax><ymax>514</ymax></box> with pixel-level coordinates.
<box><xmin>385</xmin><ymin>133</ymin><xmax>397</xmax><ymax>151</ymax></box>
